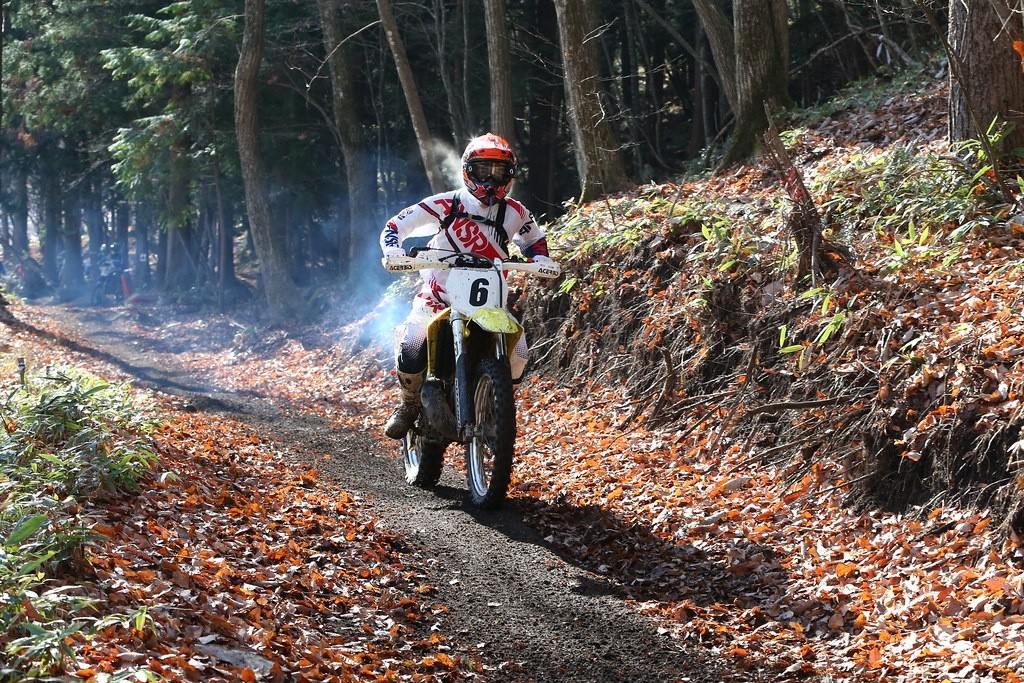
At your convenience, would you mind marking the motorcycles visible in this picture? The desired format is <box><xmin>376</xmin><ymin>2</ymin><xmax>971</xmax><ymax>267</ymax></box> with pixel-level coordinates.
<box><xmin>375</xmin><ymin>257</ymin><xmax>566</xmax><ymax>521</ymax></box>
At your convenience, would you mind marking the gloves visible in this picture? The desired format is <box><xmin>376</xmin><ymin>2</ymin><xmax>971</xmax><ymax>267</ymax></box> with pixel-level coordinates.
<box><xmin>383</xmin><ymin>247</ymin><xmax>406</xmax><ymax>258</ymax></box>
<box><xmin>532</xmin><ymin>254</ymin><xmax>553</xmax><ymax>262</ymax></box>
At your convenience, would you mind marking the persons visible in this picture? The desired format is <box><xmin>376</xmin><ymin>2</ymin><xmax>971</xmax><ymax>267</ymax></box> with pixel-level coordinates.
<box><xmin>379</xmin><ymin>133</ymin><xmax>549</xmax><ymax>439</ymax></box>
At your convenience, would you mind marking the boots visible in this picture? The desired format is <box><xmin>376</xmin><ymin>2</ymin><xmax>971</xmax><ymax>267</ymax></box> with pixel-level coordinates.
<box><xmin>384</xmin><ymin>366</ymin><xmax>428</xmax><ymax>440</ymax></box>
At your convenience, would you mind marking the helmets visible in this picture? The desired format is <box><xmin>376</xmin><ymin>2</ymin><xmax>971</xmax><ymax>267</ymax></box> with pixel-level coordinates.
<box><xmin>461</xmin><ymin>132</ymin><xmax>517</xmax><ymax>206</ymax></box>
